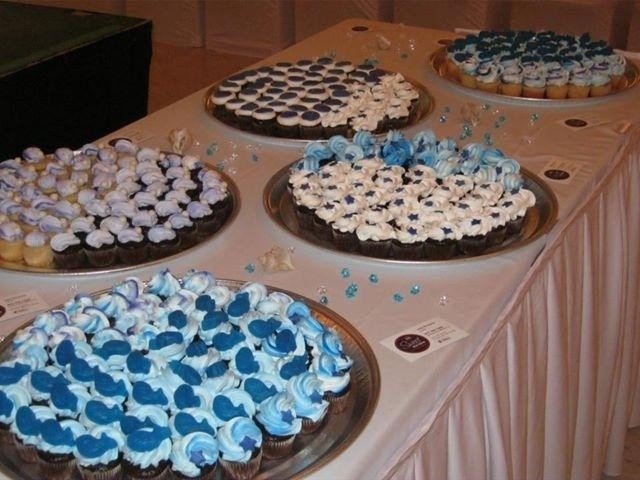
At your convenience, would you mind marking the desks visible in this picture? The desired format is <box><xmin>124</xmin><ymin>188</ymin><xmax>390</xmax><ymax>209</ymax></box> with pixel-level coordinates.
<box><xmin>0</xmin><ymin>0</ymin><xmax>155</xmax><ymax>163</ymax></box>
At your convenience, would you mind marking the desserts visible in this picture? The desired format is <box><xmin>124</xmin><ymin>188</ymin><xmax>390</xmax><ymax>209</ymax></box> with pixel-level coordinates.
<box><xmin>0</xmin><ymin>267</ymin><xmax>355</xmax><ymax>479</ymax></box>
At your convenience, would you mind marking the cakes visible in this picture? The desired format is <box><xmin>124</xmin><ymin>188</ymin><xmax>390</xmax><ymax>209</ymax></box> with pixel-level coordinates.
<box><xmin>288</xmin><ymin>128</ymin><xmax>536</xmax><ymax>257</ymax></box>
<box><xmin>439</xmin><ymin>28</ymin><xmax>627</xmax><ymax>100</ymax></box>
<box><xmin>0</xmin><ymin>138</ymin><xmax>232</xmax><ymax>269</ymax></box>
<box><xmin>208</xmin><ymin>58</ymin><xmax>420</xmax><ymax>138</ymax></box>
